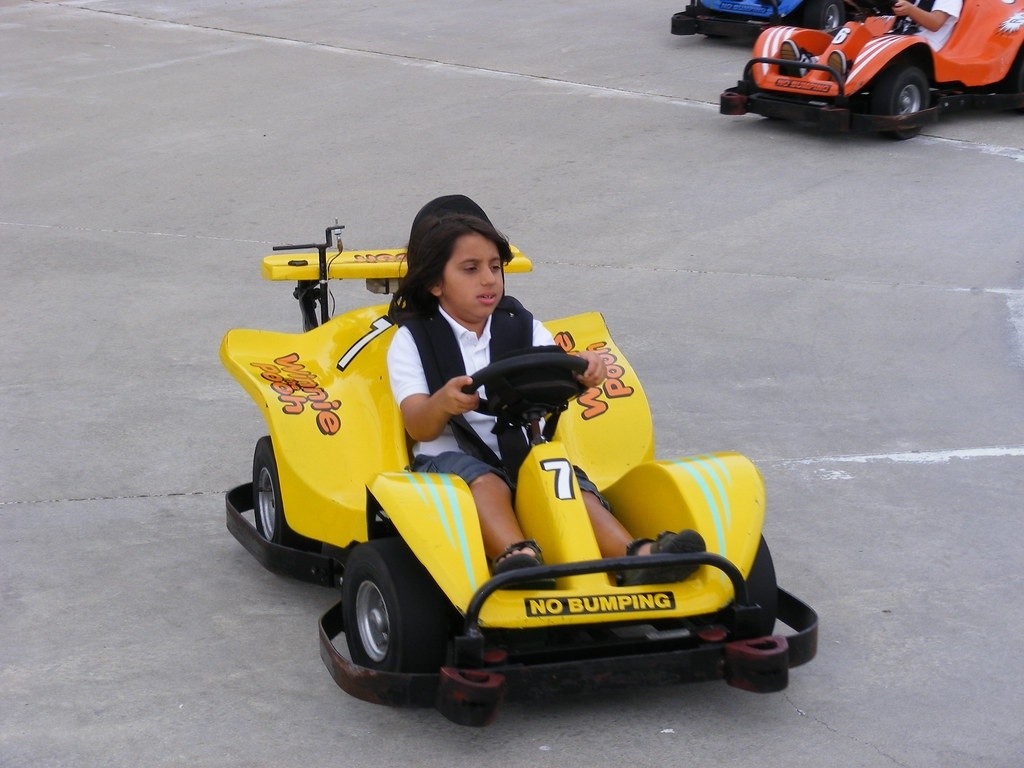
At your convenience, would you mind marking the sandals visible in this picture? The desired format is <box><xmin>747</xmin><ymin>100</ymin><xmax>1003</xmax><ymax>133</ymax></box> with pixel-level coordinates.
<box><xmin>616</xmin><ymin>530</ymin><xmax>707</xmax><ymax>586</ymax></box>
<box><xmin>492</xmin><ymin>540</ymin><xmax>556</xmax><ymax>590</ymax></box>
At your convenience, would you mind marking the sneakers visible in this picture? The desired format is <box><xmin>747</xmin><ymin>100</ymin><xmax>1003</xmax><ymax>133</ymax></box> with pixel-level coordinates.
<box><xmin>780</xmin><ymin>40</ymin><xmax>812</xmax><ymax>78</ymax></box>
<box><xmin>827</xmin><ymin>50</ymin><xmax>849</xmax><ymax>82</ymax></box>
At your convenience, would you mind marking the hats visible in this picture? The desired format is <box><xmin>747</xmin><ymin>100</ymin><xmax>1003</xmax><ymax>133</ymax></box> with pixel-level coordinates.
<box><xmin>407</xmin><ymin>195</ymin><xmax>496</xmax><ymax>268</ymax></box>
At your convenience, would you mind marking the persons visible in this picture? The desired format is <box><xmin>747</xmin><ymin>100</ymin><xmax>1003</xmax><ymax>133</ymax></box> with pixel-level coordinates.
<box><xmin>388</xmin><ymin>215</ymin><xmax>707</xmax><ymax>593</ymax></box>
<box><xmin>779</xmin><ymin>0</ymin><xmax>964</xmax><ymax>84</ymax></box>
